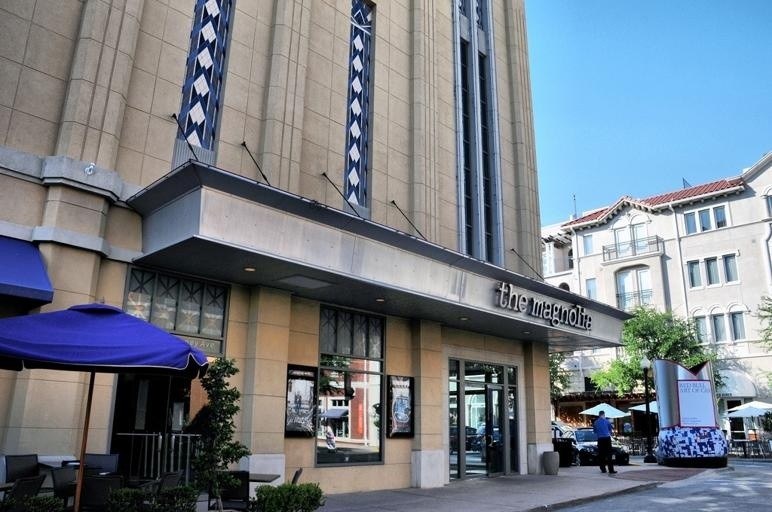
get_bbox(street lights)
[641,355,656,462]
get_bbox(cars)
[552,426,629,465]
[449,415,573,462]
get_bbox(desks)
[1,476,54,490]
[116,477,155,491]
[249,474,281,483]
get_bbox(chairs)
[52,466,77,508]
[5,454,39,482]
[4,474,48,501]
[291,468,303,485]
[139,473,177,492]
[85,454,119,476]
[86,476,122,504]
[208,470,256,511]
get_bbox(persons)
[593,411,617,474]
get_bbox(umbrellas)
[578,404,631,419]
[0,303,209,512]
[725,399,772,457]
